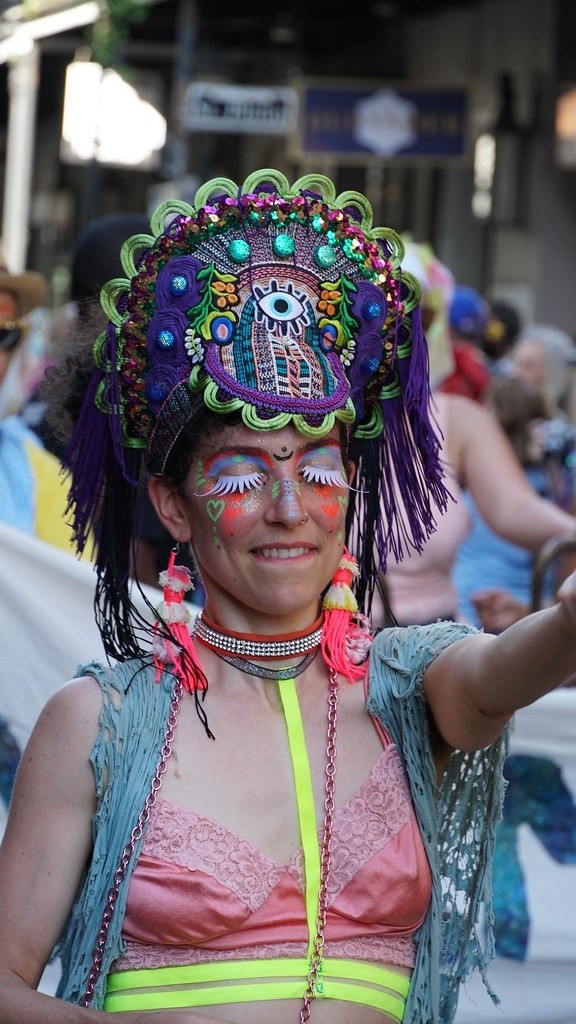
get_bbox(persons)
[1,170,576,1024]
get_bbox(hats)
[440,286,491,337]
[67,213,156,321]
[60,168,455,738]
[472,302,521,363]
[0,248,47,320]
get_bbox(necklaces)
[191,610,325,660]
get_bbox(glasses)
[0,325,25,351]
